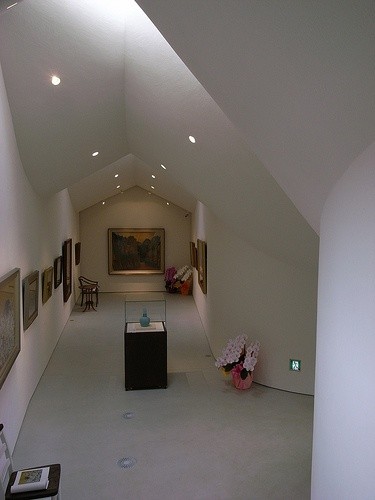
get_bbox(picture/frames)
[63,238,73,301]
[197,239,207,294]
[190,242,197,268]
[43,267,53,303]
[75,242,81,265]
[55,256,63,287]
[108,228,165,274]
[0,268,21,390]
[23,271,38,329]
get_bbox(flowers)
[173,265,192,287]
[164,267,176,289]
[215,335,260,380]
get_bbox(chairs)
[79,276,99,307]
[0,424,62,500]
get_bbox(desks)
[79,284,97,312]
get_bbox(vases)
[232,371,253,389]
[169,286,176,293]
[140,306,150,327]
[179,284,190,294]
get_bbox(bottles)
[140,313,150,327]
